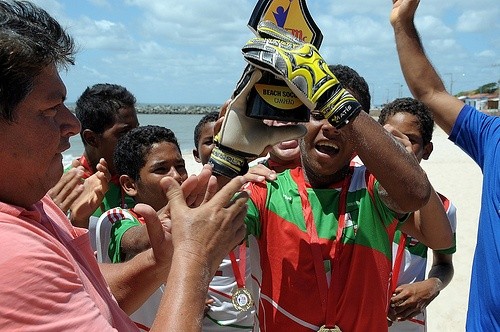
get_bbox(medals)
[318,325,342,332]
[232,289,254,312]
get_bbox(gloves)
[241,21,362,129]
[207,63,307,179]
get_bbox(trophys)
[246,0,323,122]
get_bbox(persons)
[0,0,248,332]
[46,83,301,332]
[390,0,500,332]
[373,98,457,332]
[208,20,431,332]
